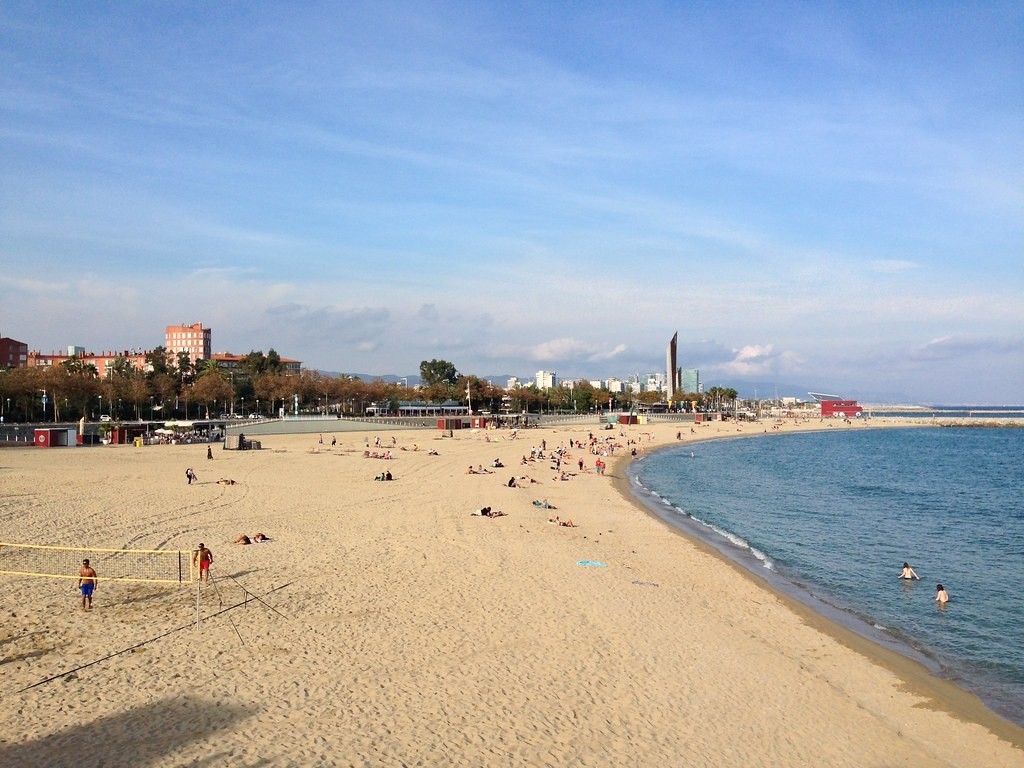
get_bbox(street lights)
[241,397,244,415]
[150,396,153,421]
[65,398,67,410]
[43,395,48,425]
[98,396,102,414]
[7,398,11,413]
[256,400,259,412]
[318,397,322,412]
[282,397,284,408]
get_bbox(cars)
[100,415,112,422]
[249,413,262,420]
[220,413,244,420]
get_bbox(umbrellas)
[155,428,174,434]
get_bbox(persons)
[898,562,920,581]
[732,416,871,432]
[936,583,949,602]
[193,543,213,582]
[219,478,234,485]
[451,427,695,527]
[186,467,194,484]
[319,433,438,482]
[208,445,212,459]
[237,533,266,544]
[78,559,97,610]
